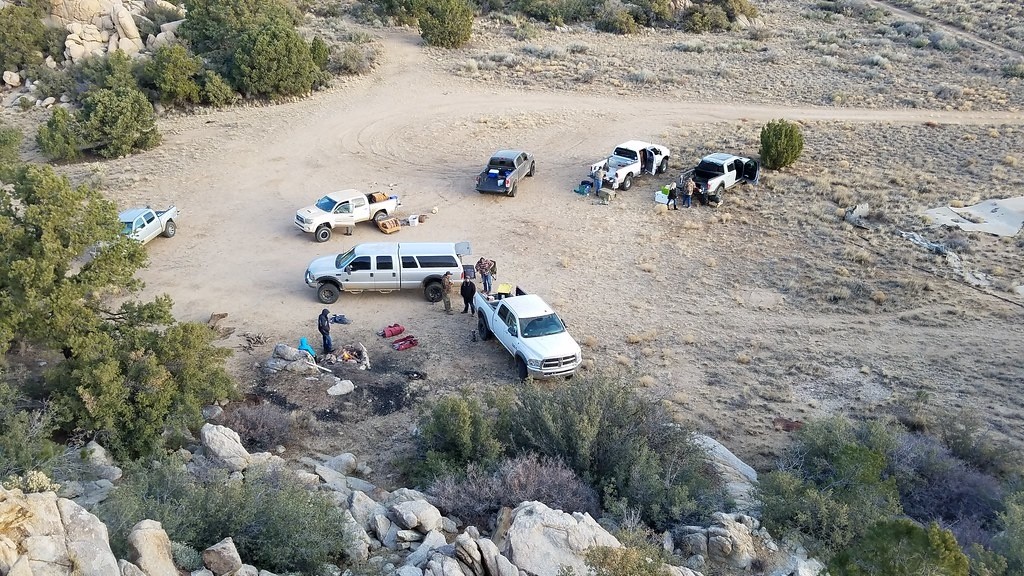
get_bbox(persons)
[318,309,333,352]
[346,226,353,235]
[594,166,605,196]
[461,276,476,317]
[441,271,454,315]
[611,173,619,197]
[666,182,678,210]
[475,257,494,294]
[682,177,696,208]
[298,337,318,365]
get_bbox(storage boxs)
[488,169,499,177]
[655,186,675,205]
[408,214,420,226]
[497,283,512,300]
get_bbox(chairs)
[490,260,497,281]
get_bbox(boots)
[668,205,670,210]
[674,205,678,210]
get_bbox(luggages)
[694,188,707,205]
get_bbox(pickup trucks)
[91,205,179,261]
[474,150,536,198]
[475,285,582,380]
[588,139,671,192]
[294,188,398,243]
[303,242,477,304]
[679,153,760,200]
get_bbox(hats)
[446,271,452,275]
[466,276,470,278]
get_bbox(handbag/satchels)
[675,198,678,205]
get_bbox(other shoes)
[472,313,474,317]
[683,204,686,206]
[481,290,487,293]
[688,206,691,209]
[461,310,467,314]
[486,291,491,294]
